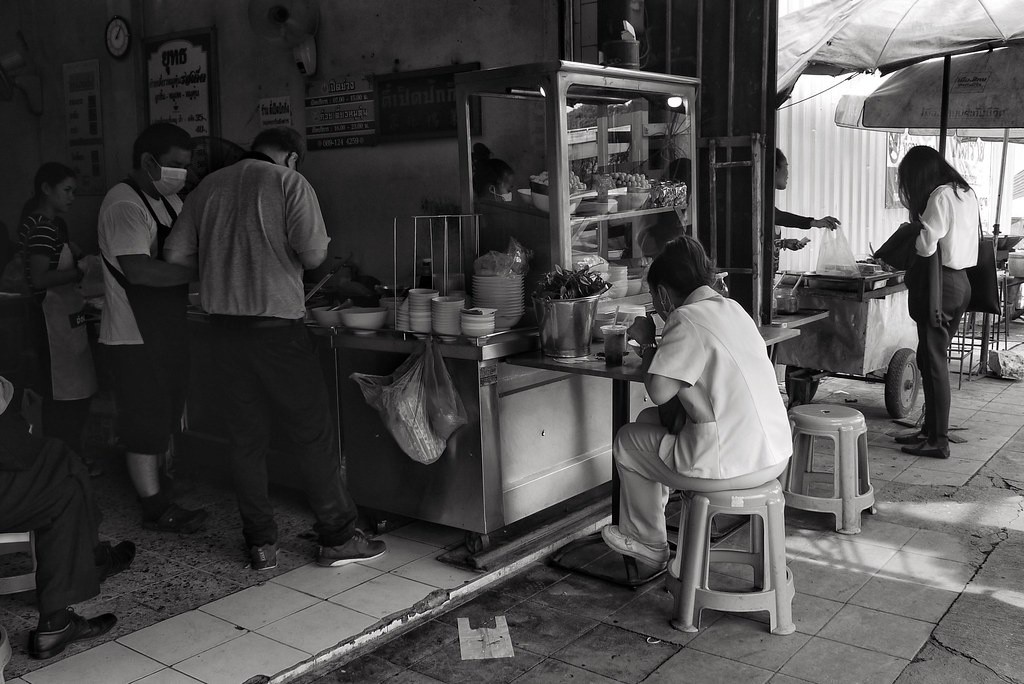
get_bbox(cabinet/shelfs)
[455,60,698,304]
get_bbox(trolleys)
[776,269,922,421]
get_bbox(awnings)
[776,0,866,111]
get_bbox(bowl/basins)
[395,180,651,344]
[342,308,388,334]
[312,306,361,327]
[628,337,663,358]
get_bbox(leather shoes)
[95,540,136,583]
[28,613,117,660]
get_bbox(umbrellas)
[807,0,1024,373]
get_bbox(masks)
[489,185,512,202]
[658,284,675,317]
[146,155,187,195]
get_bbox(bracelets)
[641,343,656,355]
[783,239,786,251]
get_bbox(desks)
[504,324,801,591]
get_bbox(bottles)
[772,287,797,318]
[602,40,640,70]
[711,271,729,297]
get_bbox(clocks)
[104,16,131,61]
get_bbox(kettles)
[600,325,626,367]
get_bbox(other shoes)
[901,440,950,458]
[895,430,928,445]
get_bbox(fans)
[0,49,41,100]
[248,0,319,75]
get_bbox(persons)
[16,160,100,473]
[773,148,841,278]
[547,154,586,182]
[602,235,793,570]
[98,121,209,533]
[471,158,515,202]
[0,377,137,660]
[895,145,979,458]
[163,128,387,571]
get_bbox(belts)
[210,319,304,329]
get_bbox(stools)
[667,480,796,636]
[779,403,878,534]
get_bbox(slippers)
[141,504,208,534]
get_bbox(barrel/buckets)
[532,295,600,358]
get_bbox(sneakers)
[601,524,670,570]
[316,528,387,567]
[249,540,281,571]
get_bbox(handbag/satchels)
[349,342,469,465]
[816,224,861,277]
[965,186,1002,316]
